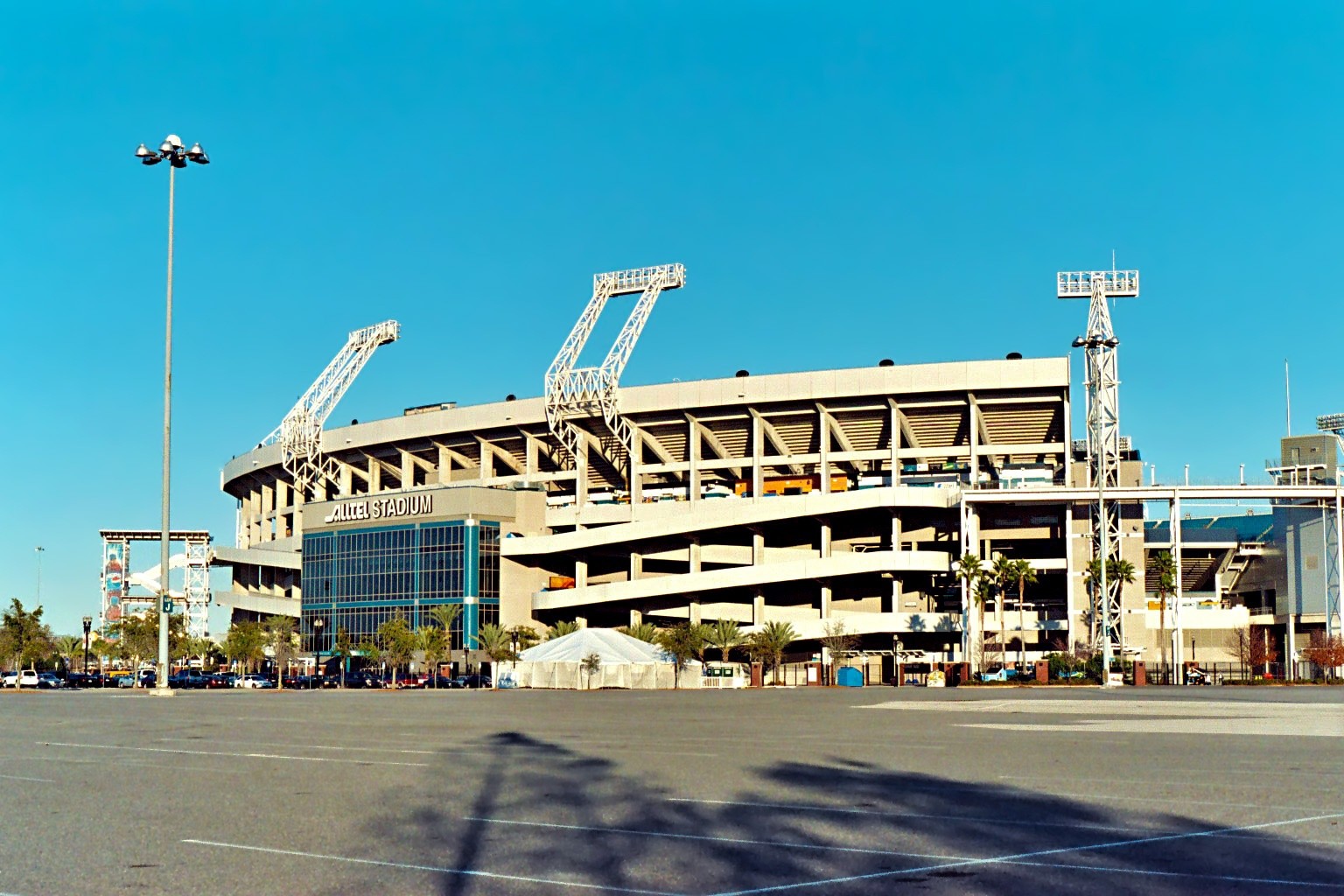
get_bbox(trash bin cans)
[702,663,742,688]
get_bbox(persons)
[1218,673,1223,683]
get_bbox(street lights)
[82,617,92,675]
[133,131,210,694]
[892,635,899,686]
[34,546,45,607]
[1072,334,1120,684]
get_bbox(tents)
[492,628,702,690]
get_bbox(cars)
[0,668,493,690]
